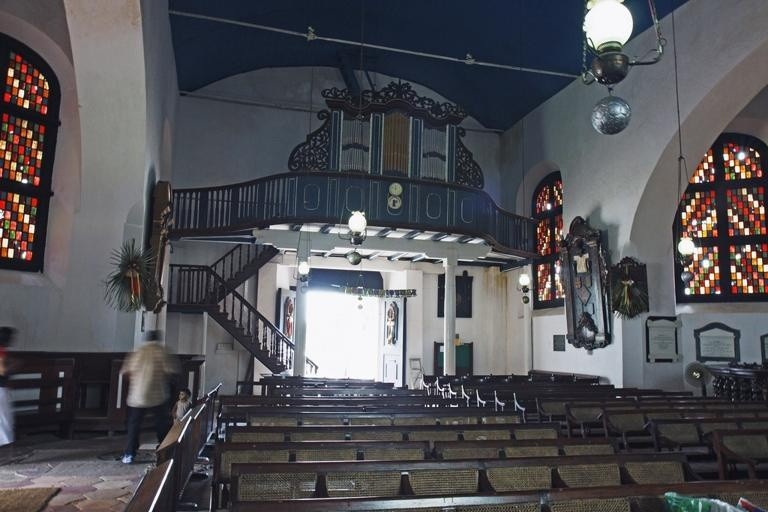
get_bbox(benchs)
[213,370,768,511]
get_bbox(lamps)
[577,0,667,137]
[337,206,371,267]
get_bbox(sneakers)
[122,454,133,464]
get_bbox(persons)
[0,326,21,446]
[120,329,182,463]
[171,388,192,425]
